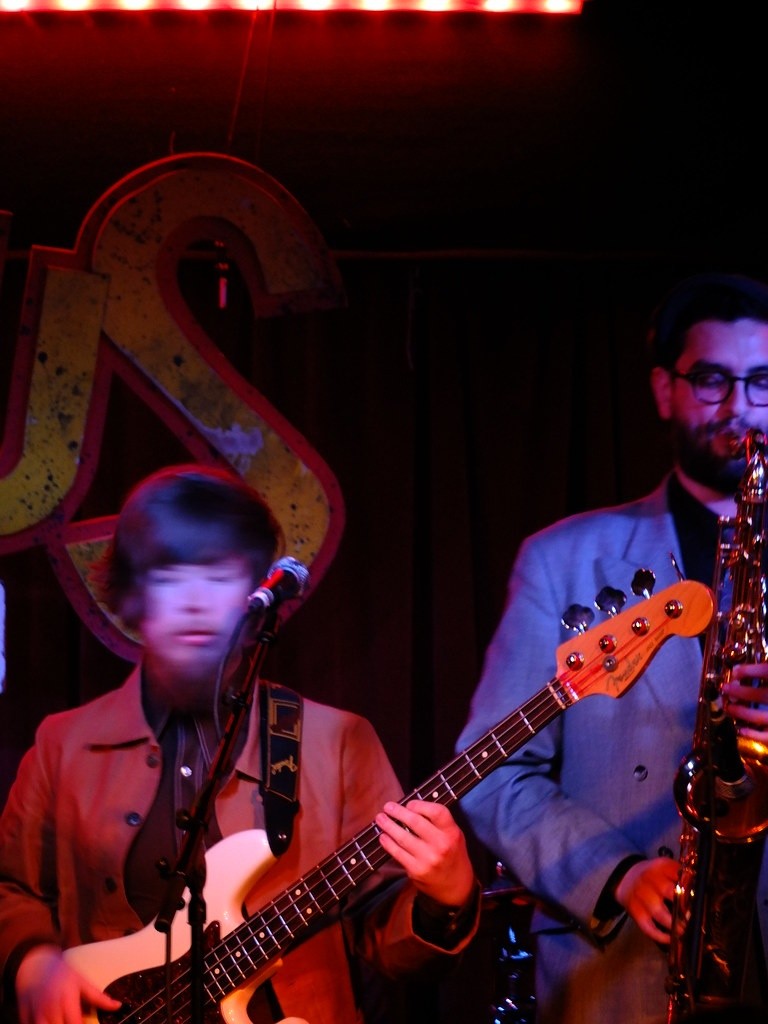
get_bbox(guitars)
[63,553,717,1024]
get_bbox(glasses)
[666,369,768,407]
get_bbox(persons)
[0,460,479,1023]
[453,290,768,1023]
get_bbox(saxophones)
[659,427,766,1023]
